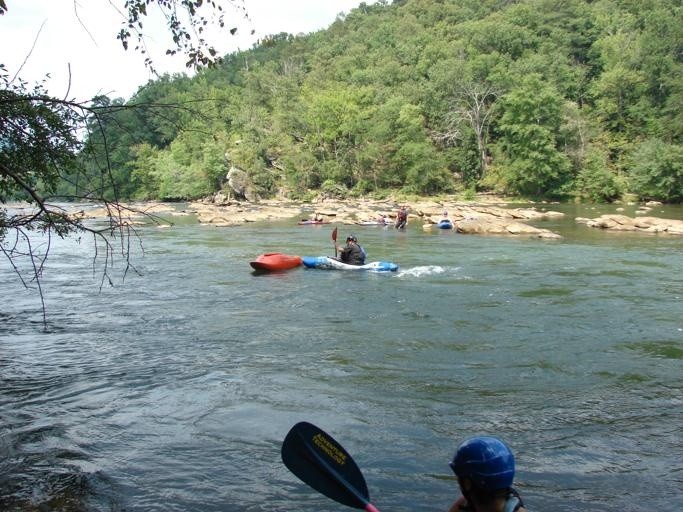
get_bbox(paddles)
[332,226,337,257]
[281,421,379,512]
[302,219,309,221]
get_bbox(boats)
[251,253,304,272]
[358,217,394,226]
[298,220,332,225]
[304,255,398,273]
[438,223,453,230]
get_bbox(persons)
[394,206,408,229]
[446,436,528,512]
[376,214,388,224]
[440,211,454,228]
[315,213,322,221]
[336,235,366,266]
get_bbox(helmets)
[347,235,357,242]
[449,436,515,490]
